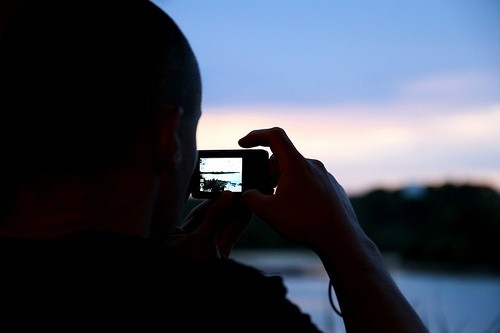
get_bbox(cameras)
[192,149,273,199]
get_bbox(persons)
[0,0,430,333]
